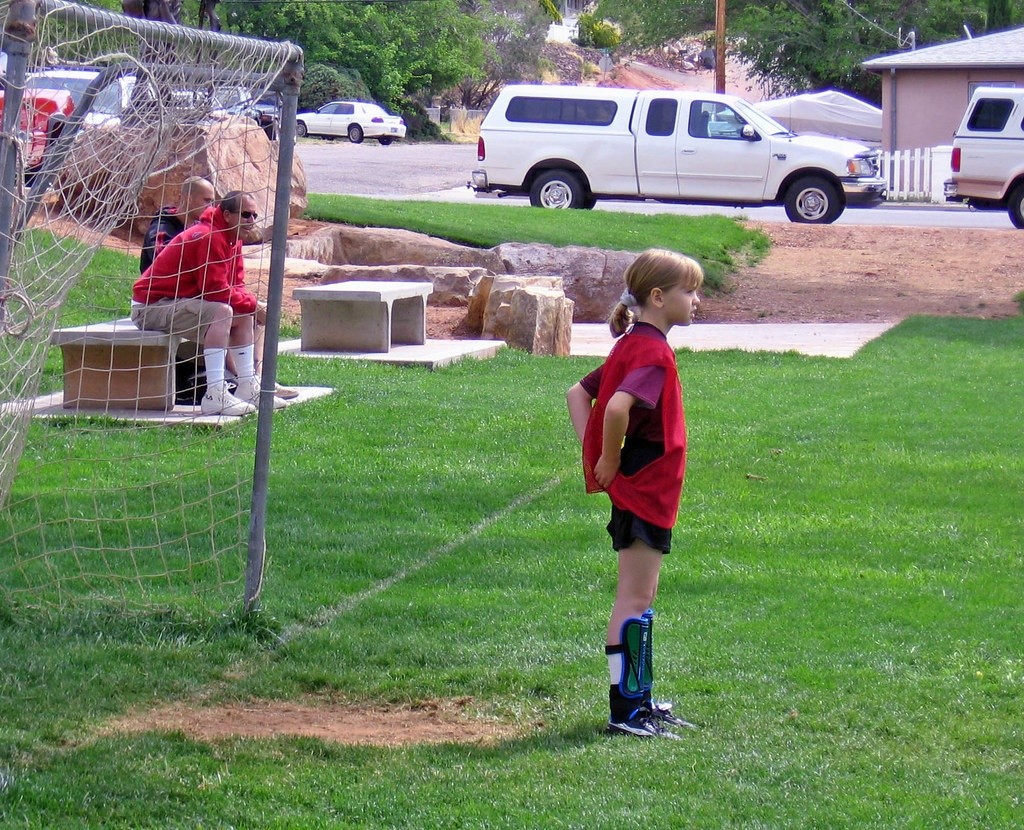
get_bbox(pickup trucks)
[943,86,1024,229]
[466,85,888,225]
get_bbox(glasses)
[229,210,258,220]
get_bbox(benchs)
[50,314,205,412]
[291,280,433,353]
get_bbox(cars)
[296,101,406,145]
[1,71,283,171]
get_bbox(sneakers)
[605,703,693,741]
[200,388,256,417]
[234,388,288,409]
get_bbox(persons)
[565,249,705,745]
[130,190,287,415]
[139,175,299,399]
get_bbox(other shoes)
[276,383,300,399]
